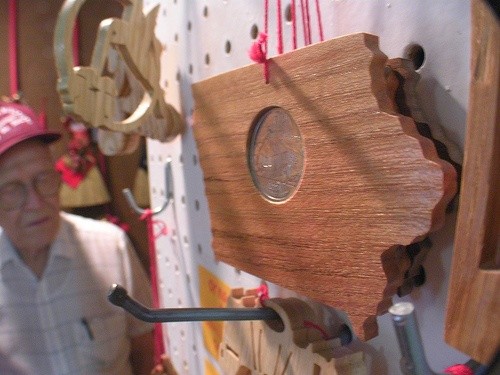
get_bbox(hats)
[0,100,64,156]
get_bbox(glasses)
[1,171,64,210]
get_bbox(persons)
[0,100,171,375]
